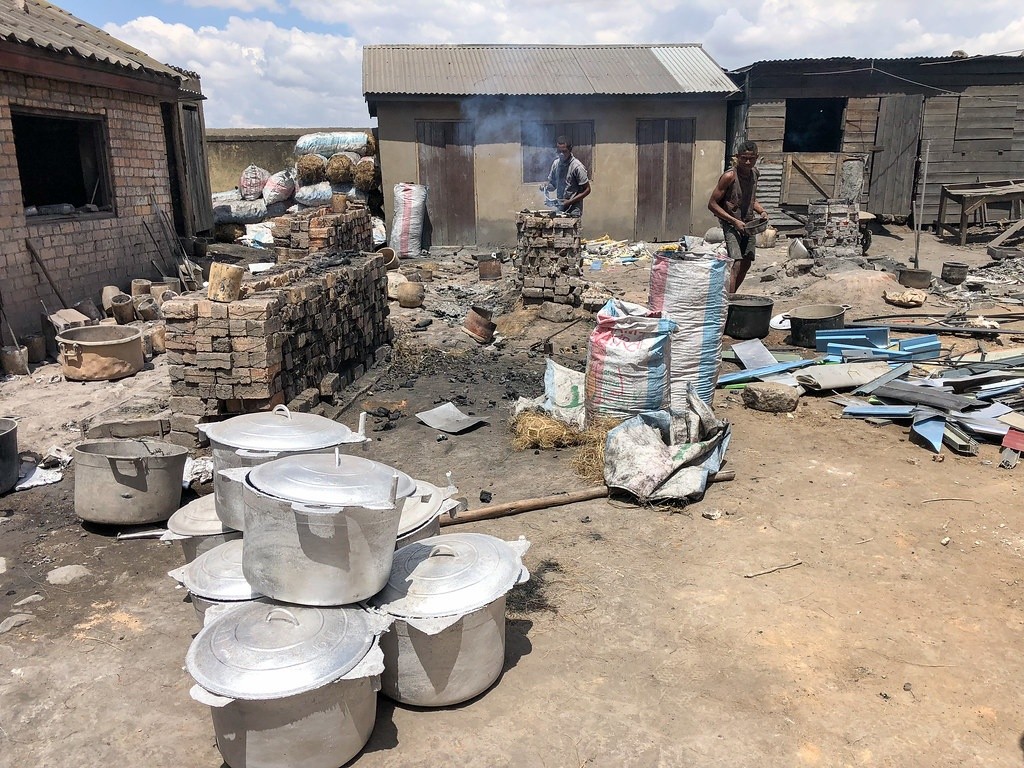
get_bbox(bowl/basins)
[743,218,768,234]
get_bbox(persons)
[538,134,591,218]
[707,141,769,294]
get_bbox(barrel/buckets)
[941,262,969,285]
[195,403,373,532]
[159,492,243,564]
[728,293,775,338]
[54,325,144,380]
[73,437,188,525]
[782,304,852,347]
[0,415,20,494]
[899,269,931,288]
[185,596,382,768]
[217,448,416,606]
[395,479,461,552]
[367,533,532,706]
